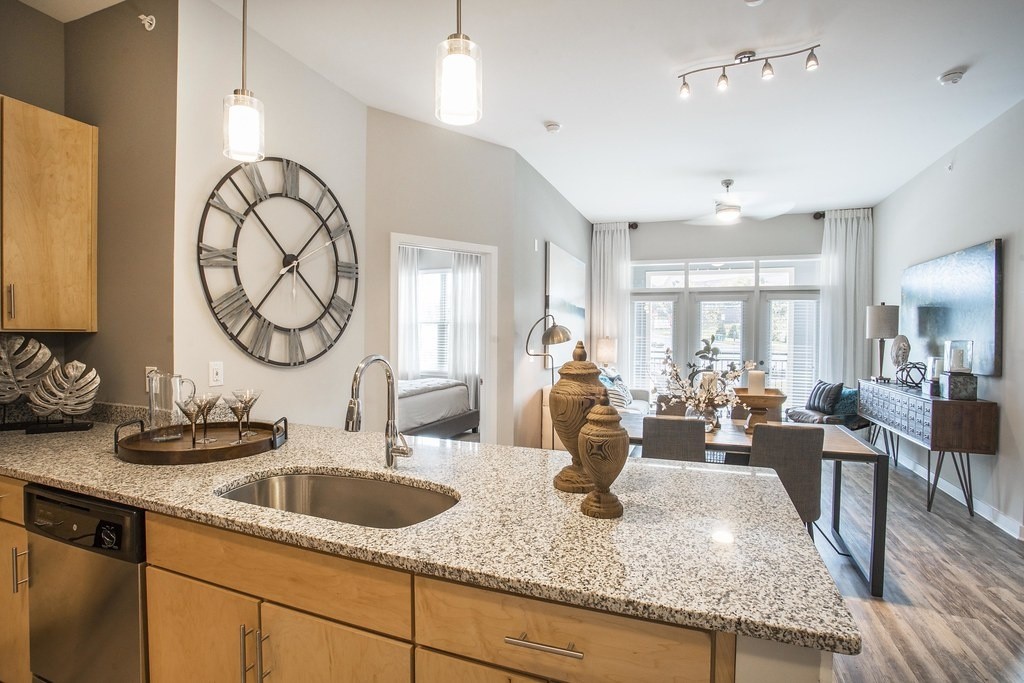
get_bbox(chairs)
[642,416,706,462]
[748,423,825,542]
[656,395,687,416]
[785,386,876,431]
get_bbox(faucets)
[344,353,413,469]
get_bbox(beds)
[398,378,479,438]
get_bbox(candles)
[747,370,765,394]
[934,360,943,377]
[952,349,964,368]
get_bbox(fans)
[682,177,796,226]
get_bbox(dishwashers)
[11,483,148,683]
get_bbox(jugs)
[146,370,196,442]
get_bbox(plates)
[891,335,910,367]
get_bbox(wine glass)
[187,393,223,444]
[174,398,211,448]
[232,390,263,437]
[223,396,255,446]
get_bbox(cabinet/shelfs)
[0,94,98,332]
[0,478,736,683]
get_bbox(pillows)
[606,379,633,409]
[804,379,844,416]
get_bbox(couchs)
[542,383,650,452]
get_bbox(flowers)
[645,333,757,411]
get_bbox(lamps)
[222,0,265,162]
[677,44,821,97]
[434,0,482,126]
[596,336,618,367]
[866,302,899,383]
[716,205,740,221]
[526,314,572,450]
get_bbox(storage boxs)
[921,381,939,396]
[939,374,977,400]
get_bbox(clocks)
[197,156,359,365]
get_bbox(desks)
[856,379,998,517]
[617,413,889,598]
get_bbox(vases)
[685,405,717,432]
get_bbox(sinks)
[210,462,462,530]
